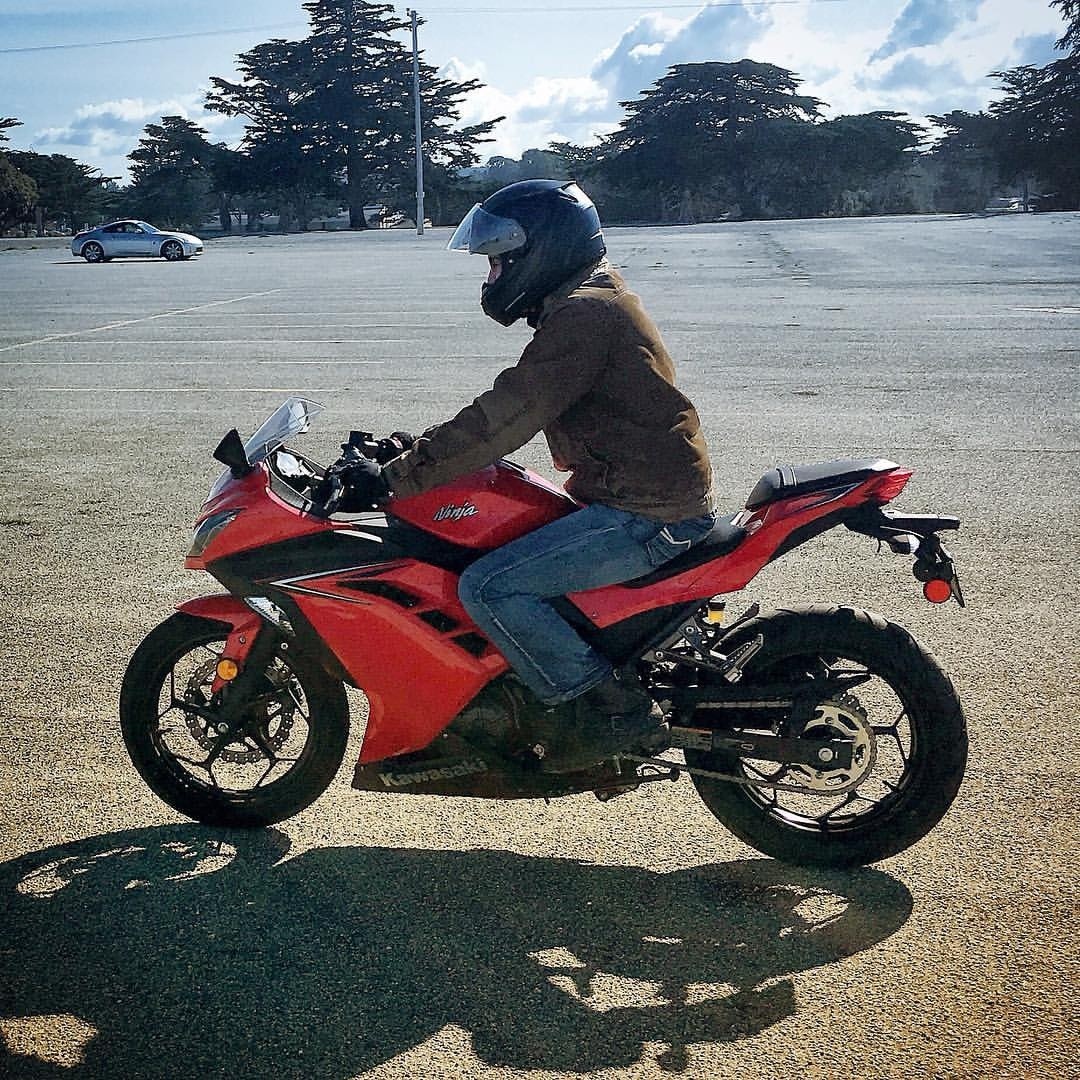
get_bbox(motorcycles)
[116,394,970,873]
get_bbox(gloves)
[343,459,388,500]
[379,431,417,463]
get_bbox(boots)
[531,669,672,774]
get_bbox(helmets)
[472,183,607,326]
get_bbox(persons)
[323,178,717,774]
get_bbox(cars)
[983,197,1025,214]
[47,230,66,237]
[72,220,204,264]
